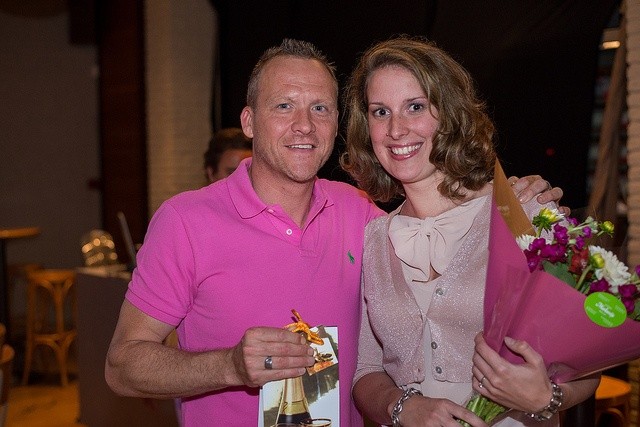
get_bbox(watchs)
[525,382,564,422]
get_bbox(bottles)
[275,376,313,427]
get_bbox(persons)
[204,128,253,184]
[340,40,602,427]
[105,42,571,427]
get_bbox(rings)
[265,356,272,369]
[479,376,486,387]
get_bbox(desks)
[0,226,42,386]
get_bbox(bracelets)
[391,387,423,427]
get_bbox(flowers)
[458,207,640,426]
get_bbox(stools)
[20,264,80,386]
